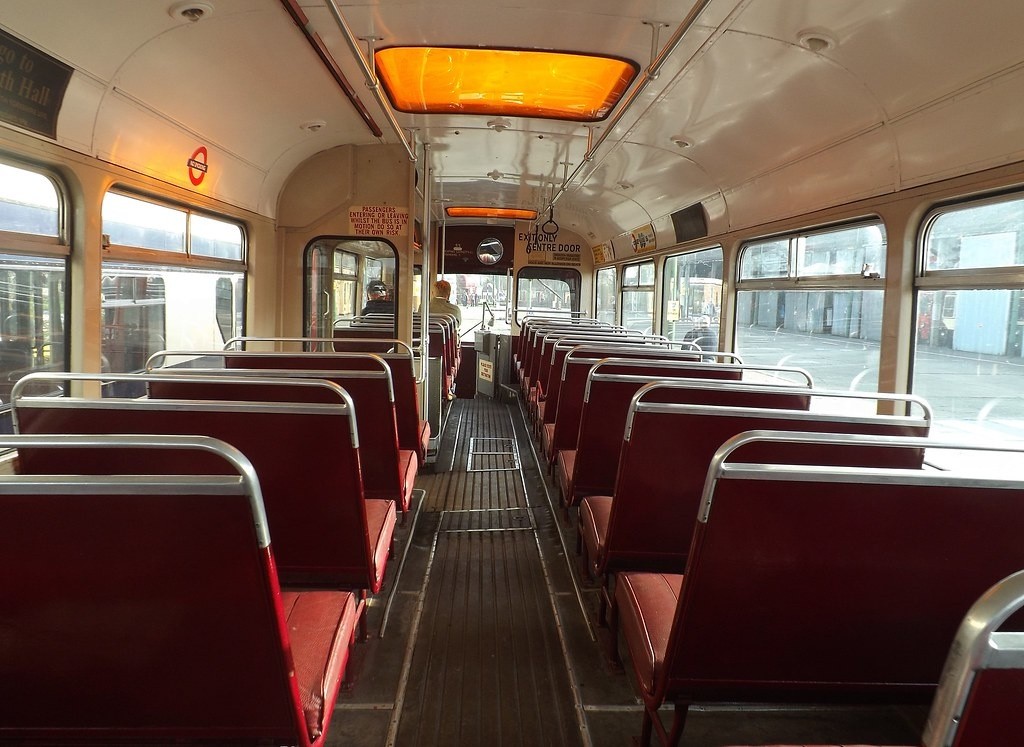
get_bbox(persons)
[361,280,395,323]
[418,279,461,328]
[681,314,719,360]
[704,300,715,323]
[462,290,477,307]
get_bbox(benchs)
[0,313,460,747]
[515,315,1024,747]
